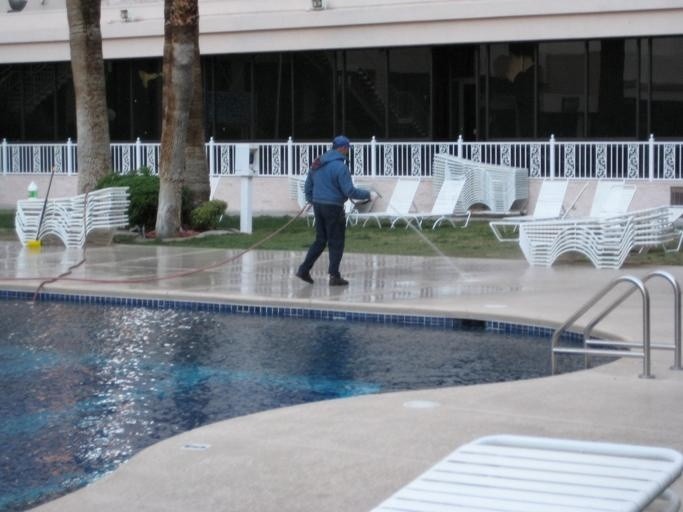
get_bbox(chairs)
[370,434,683,512]
[289,152,529,231]
[489,176,683,269]
[14,187,131,248]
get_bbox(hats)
[333,136,352,147]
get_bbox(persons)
[297,135,380,285]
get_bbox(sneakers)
[329,277,348,286]
[296,271,313,284]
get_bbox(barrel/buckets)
[27,180,38,198]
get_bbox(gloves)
[369,191,379,201]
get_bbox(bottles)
[27,182,38,201]
